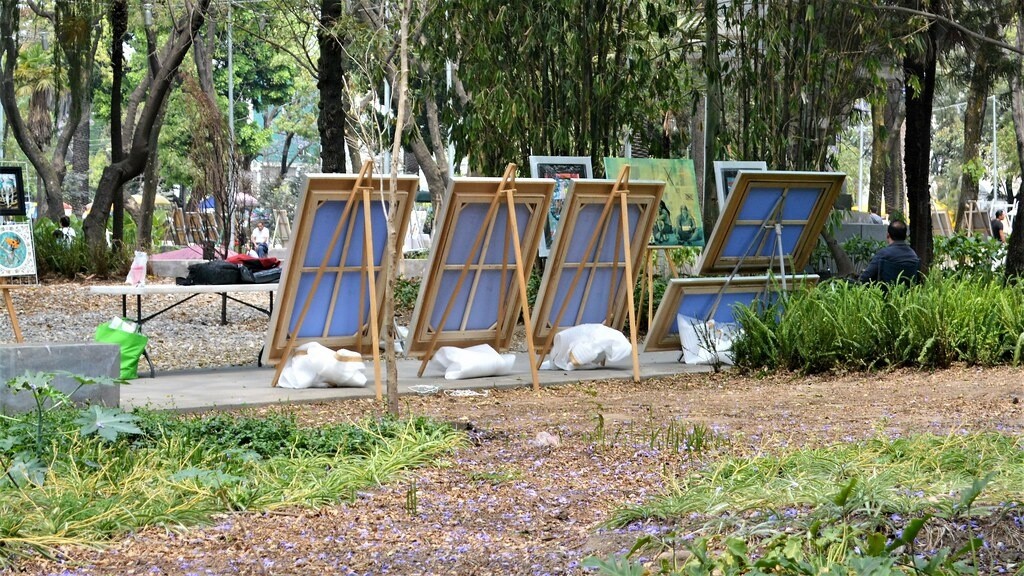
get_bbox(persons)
[250,222,269,258]
[862,219,920,286]
[59,216,77,244]
[991,209,1006,243]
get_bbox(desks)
[90,284,280,379]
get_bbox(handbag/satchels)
[95,317,148,380]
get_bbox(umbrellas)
[196,191,260,208]
